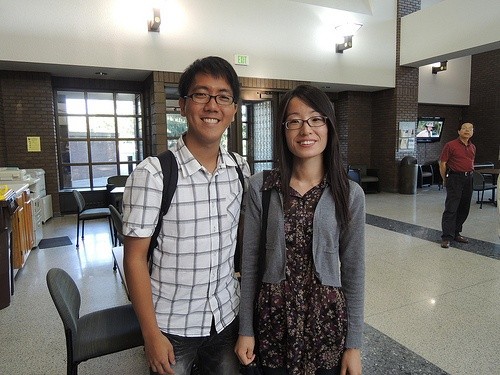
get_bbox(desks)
[475,169,499,208]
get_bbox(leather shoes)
[455,236,468,243]
[441,240,450,248]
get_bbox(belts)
[449,171,474,176]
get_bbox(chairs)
[73,177,125,270]
[472,170,497,209]
[419,164,434,188]
[45,268,145,375]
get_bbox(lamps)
[334,22,363,54]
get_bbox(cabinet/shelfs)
[0,185,35,309]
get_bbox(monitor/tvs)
[416,117,445,142]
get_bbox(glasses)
[283,115,329,130]
[185,93,237,106]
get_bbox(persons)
[234,85,366,375]
[417,122,435,137]
[439,121,476,248]
[122,57,251,375]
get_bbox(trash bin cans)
[399,155,418,194]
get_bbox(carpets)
[38,236,73,248]
